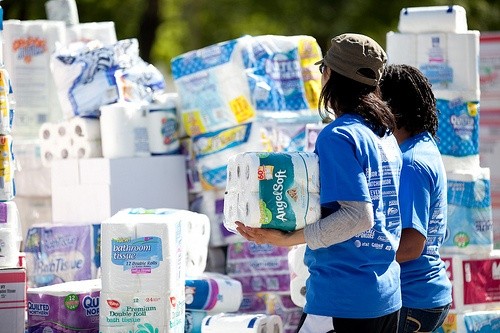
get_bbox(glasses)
[319,65,329,77]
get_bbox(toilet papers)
[0,0,499,333]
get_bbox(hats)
[314,33,388,86]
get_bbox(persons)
[235,33,404,333]
[381,64,453,333]
